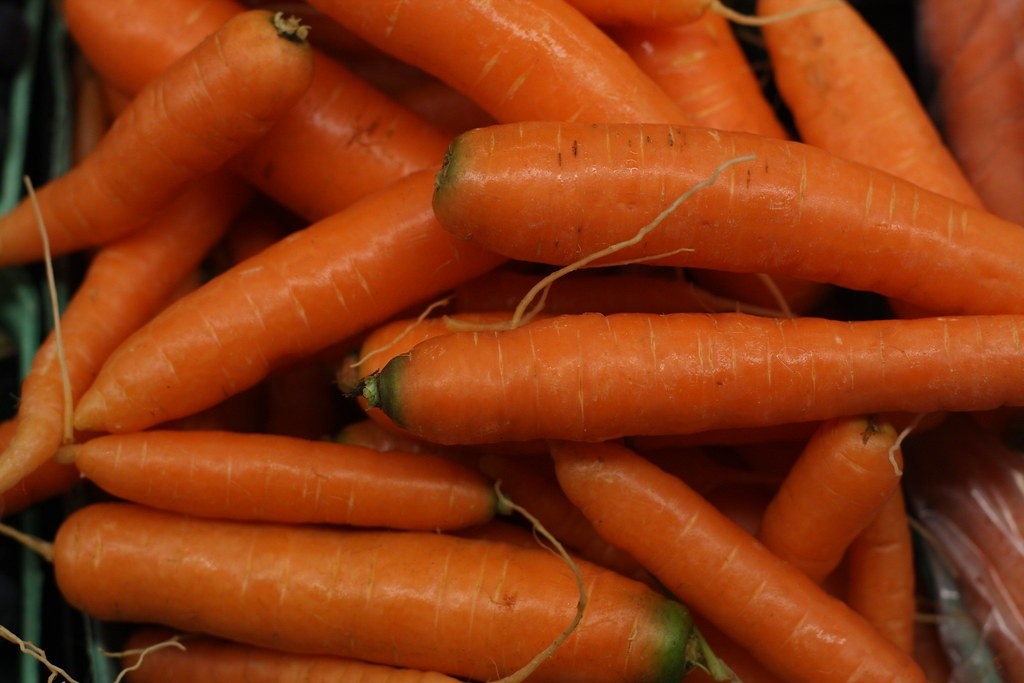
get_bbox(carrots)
[2,0,1024,681]
